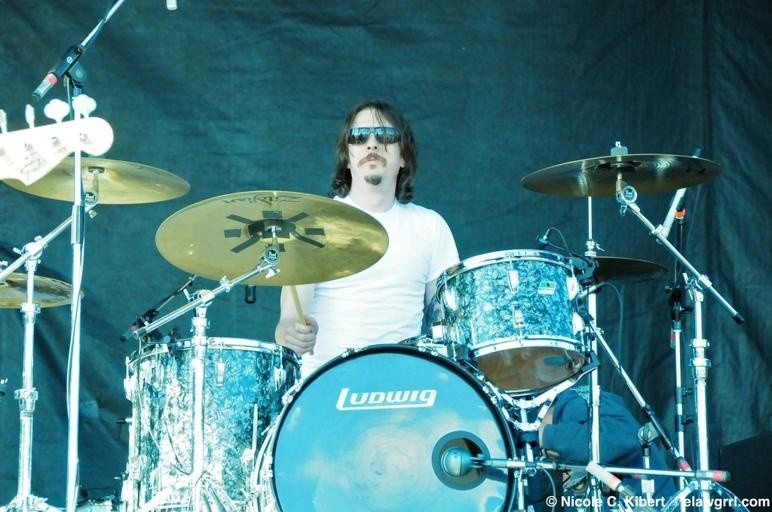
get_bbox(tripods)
[135,340,242,512]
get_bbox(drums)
[251,345,522,511]
[434,249,591,397]
[122,337,301,510]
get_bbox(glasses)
[346,126,397,144]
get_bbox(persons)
[273,99,462,380]
[537,386,677,511]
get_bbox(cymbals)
[0,156,191,205]
[522,153,722,196]
[583,256,667,283]
[155,190,389,287]
[0,270,84,308]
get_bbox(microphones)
[583,457,662,512]
[656,188,688,247]
